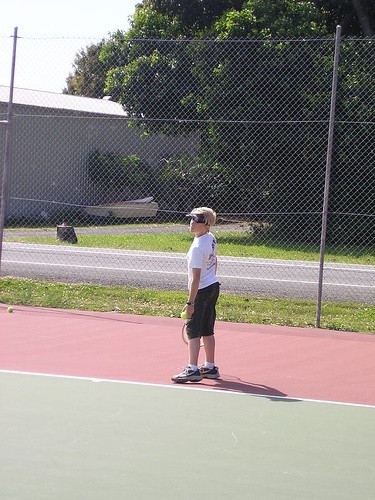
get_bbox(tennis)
[182,312,187,319]
[7,307,13,313]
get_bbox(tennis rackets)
[182,319,204,348]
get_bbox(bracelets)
[186,302,195,306]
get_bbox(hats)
[186,207,216,226]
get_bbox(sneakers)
[199,366,220,378]
[171,366,203,383]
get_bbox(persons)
[171,207,222,383]
